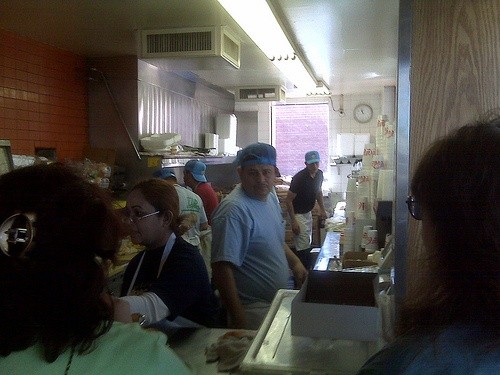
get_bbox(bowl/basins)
[140,132,181,152]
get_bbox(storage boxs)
[290,270,382,343]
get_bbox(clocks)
[353,103,373,124]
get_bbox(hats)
[305,151,320,164]
[184,160,207,182]
[151,168,177,179]
[237,143,281,177]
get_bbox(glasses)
[406,195,439,220]
[122,210,160,224]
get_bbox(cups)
[343,114,393,253]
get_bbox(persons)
[183,160,219,221]
[0,155,194,375]
[286,150,327,271]
[210,142,307,330]
[273,165,283,185]
[105,177,223,328]
[150,169,207,256]
[359,108,500,375]
